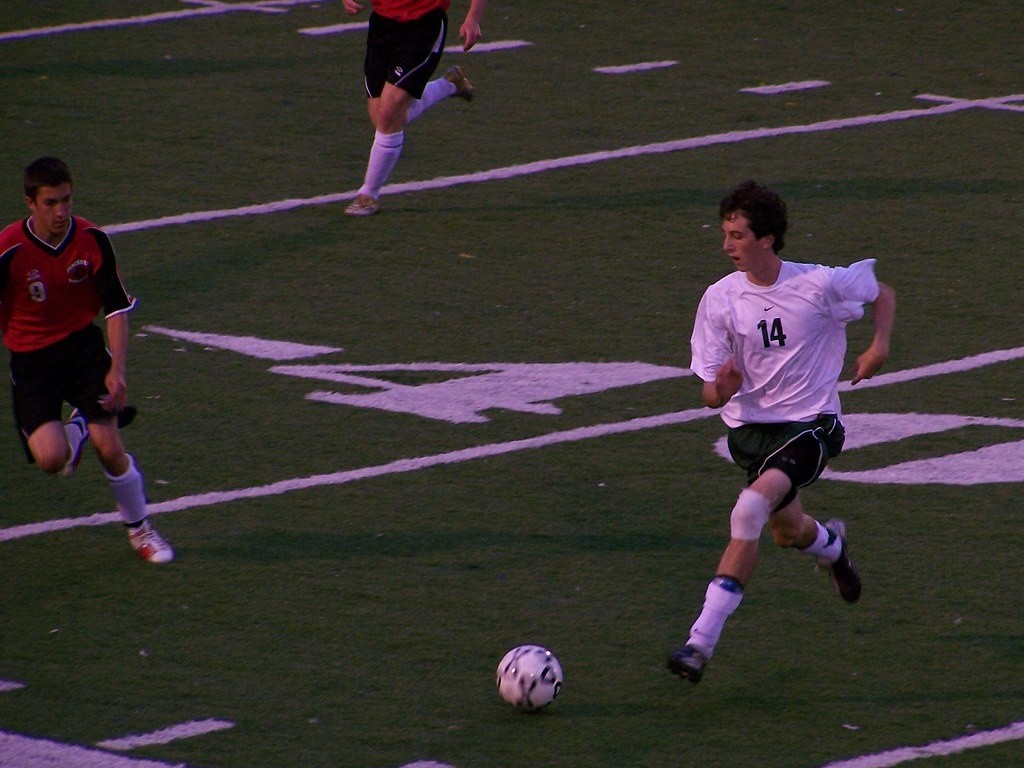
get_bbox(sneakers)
[342,193,381,216]
[127,518,173,564]
[664,645,709,687]
[62,407,90,478]
[814,517,861,604]
[443,63,474,104]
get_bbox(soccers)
[497,644,564,711]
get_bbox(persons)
[345,1,488,216]
[663,180,896,684]
[0,158,176,562]
